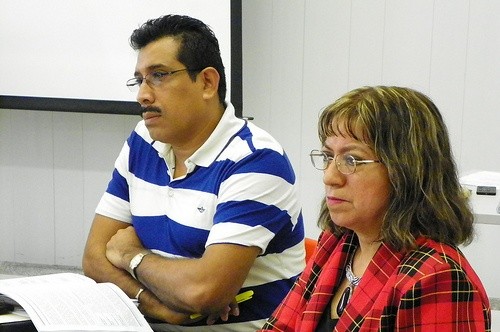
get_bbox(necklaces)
[335,241,363,316]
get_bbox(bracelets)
[132,285,147,302]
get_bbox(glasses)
[126,66,203,90]
[309,147,392,175]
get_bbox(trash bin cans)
[450,170,500,312]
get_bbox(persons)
[257,85,492,331]
[82,14,306,332]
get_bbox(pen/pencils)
[188,289,255,321]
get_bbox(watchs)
[128,250,155,281]
